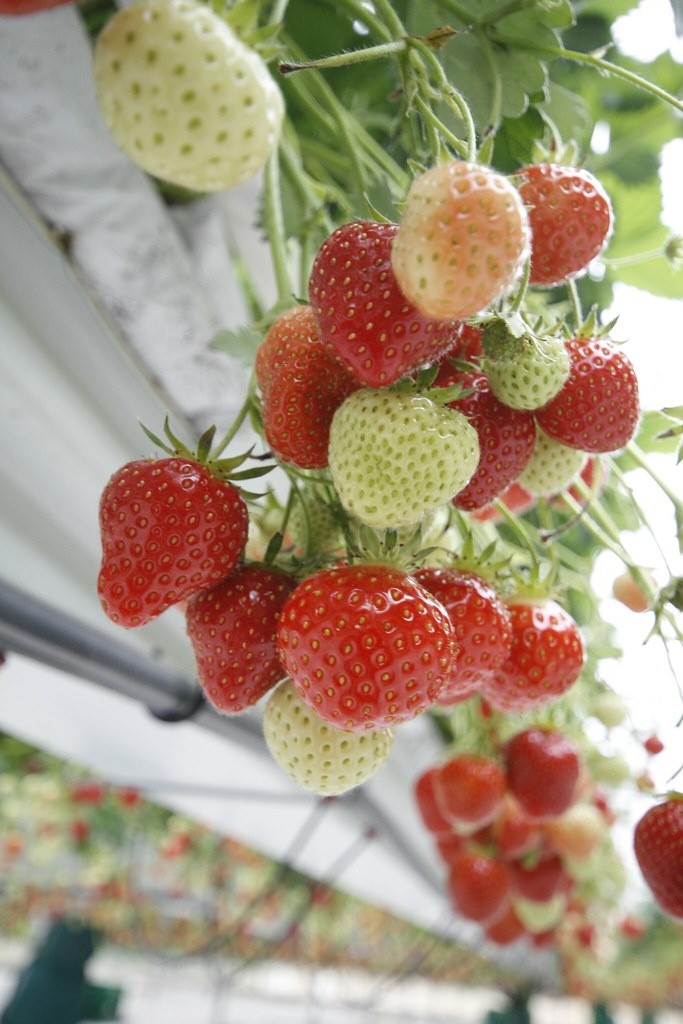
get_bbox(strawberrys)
[91,0,682,940]
